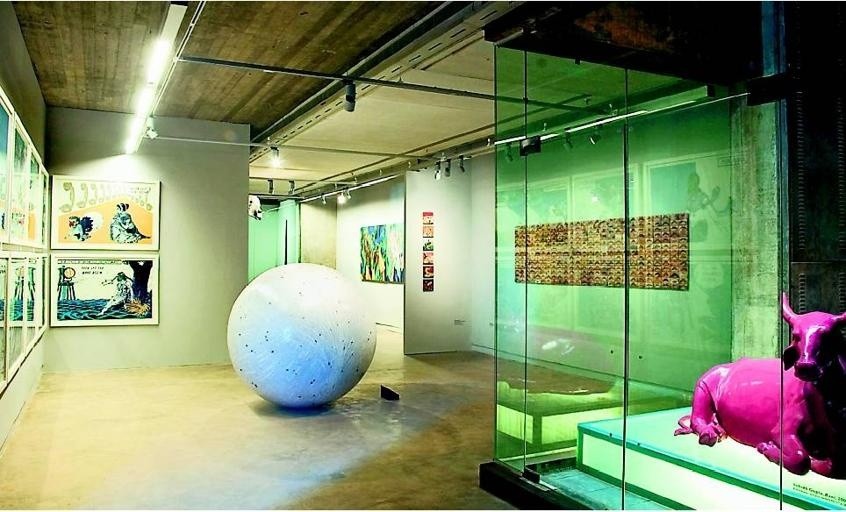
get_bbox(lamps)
[434,153,467,181]
[266,179,296,195]
[344,80,357,112]
[562,124,603,152]
[504,141,514,164]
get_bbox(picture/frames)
[1,251,50,394]
[50,175,161,252]
[0,86,51,251]
[50,250,160,328]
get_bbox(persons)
[109,201,144,246]
[95,271,135,317]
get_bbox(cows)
[674,291,846,479]
